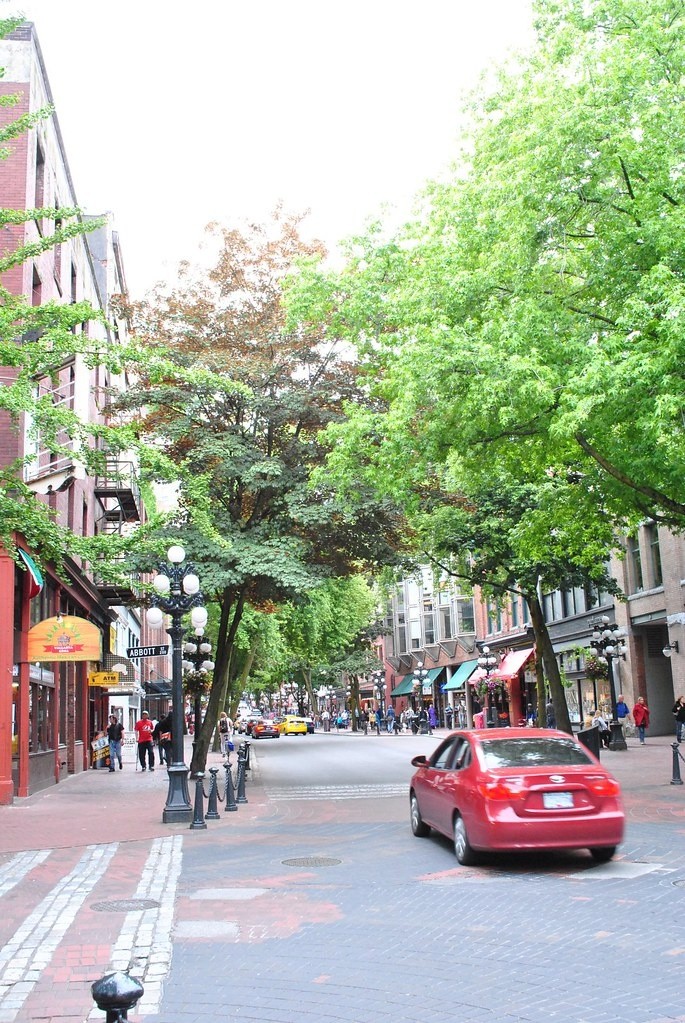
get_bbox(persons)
[159,711,173,766]
[672,696,685,743]
[585,710,612,748]
[303,699,465,734]
[218,712,233,757]
[546,699,555,728]
[134,711,154,772]
[616,695,631,739]
[152,717,159,727]
[154,714,167,766]
[107,714,124,772]
[633,697,650,744]
[525,703,537,724]
[473,698,482,713]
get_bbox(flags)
[18,548,42,599]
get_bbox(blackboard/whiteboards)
[121,731,138,764]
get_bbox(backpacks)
[219,719,228,733]
[594,718,603,732]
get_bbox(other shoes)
[142,767,146,772]
[640,741,646,745]
[120,763,123,770]
[678,740,681,743]
[604,742,609,748]
[223,754,225,757]
[109,767,116,772]
[149,766,154,771]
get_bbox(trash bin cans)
[577,725,600,760]
[411,716,418,732]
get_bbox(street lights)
[145,545,213,824]
[590,615,628,752]
[477,646,498,728]
[410,661,429,734]
[372,671,387,730]
[325,685,336,728]
[181,624,214,771]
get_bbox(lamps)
[662,641,679,657]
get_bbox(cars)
[232,700,285,736]
[303,717,315,734]
[251,719,280,739]
[277,715,308,736]
[409,727,626,867]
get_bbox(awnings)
[443,660,479,690]
[390,674,414,696]
[498,648,534,680]
[423,667,444,687]
[403,683,414,694]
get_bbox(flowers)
[412,683,422,697]
[584,657,608,679]
[473,675,502,697]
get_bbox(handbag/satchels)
[673,703,679,718]
[387,715,393,721]
